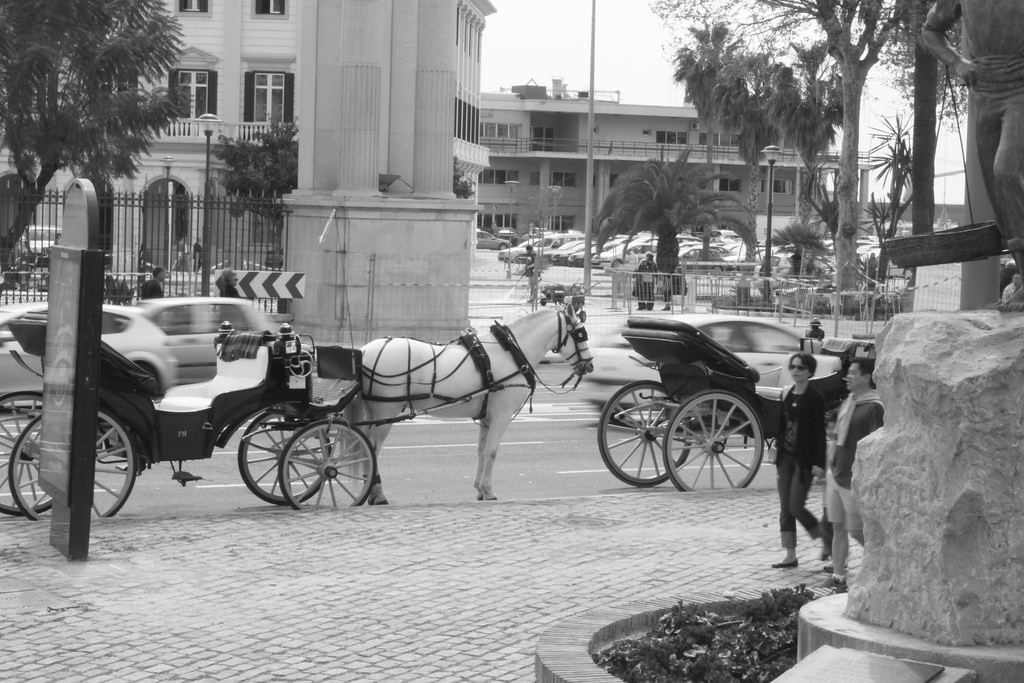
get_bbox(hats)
[809,318,822,326]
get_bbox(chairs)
[730,331,751,352]
[669,276,696,312]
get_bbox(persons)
[791,251,816,275]
[758,256,766,298]
[193,237,203,272]
[856,253,877,290]
[633,252,658,311]
[770,352,884,589]
[524,245,540,302]
[999,260,1021,301]
[219,270,240,298]
[141,267,166,300]
[661,273,672,311]
[921,0,1024,313]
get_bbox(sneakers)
[824,561,849,572]
[813,577,848,594]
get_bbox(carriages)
[0,291,596,525]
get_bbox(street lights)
[504,179,519,280]
[193,112,222,299]
[759,144,784,312]
[550,185,562,234]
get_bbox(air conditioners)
[690,121,700,129]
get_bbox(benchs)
[264,339,318,364]
[755,353,842,400]
[156,340,268,410]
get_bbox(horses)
[344,293,595,504]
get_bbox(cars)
[0,301,181,407]
[9,223,62,271]
[475,227,917,281]
[131,297,279,388]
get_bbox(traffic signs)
[212,268,305,299]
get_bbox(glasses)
[788,364,809,372]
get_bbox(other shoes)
[821,532,833,561]
[771,558,799,568]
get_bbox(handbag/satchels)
[769,446,778,464]
